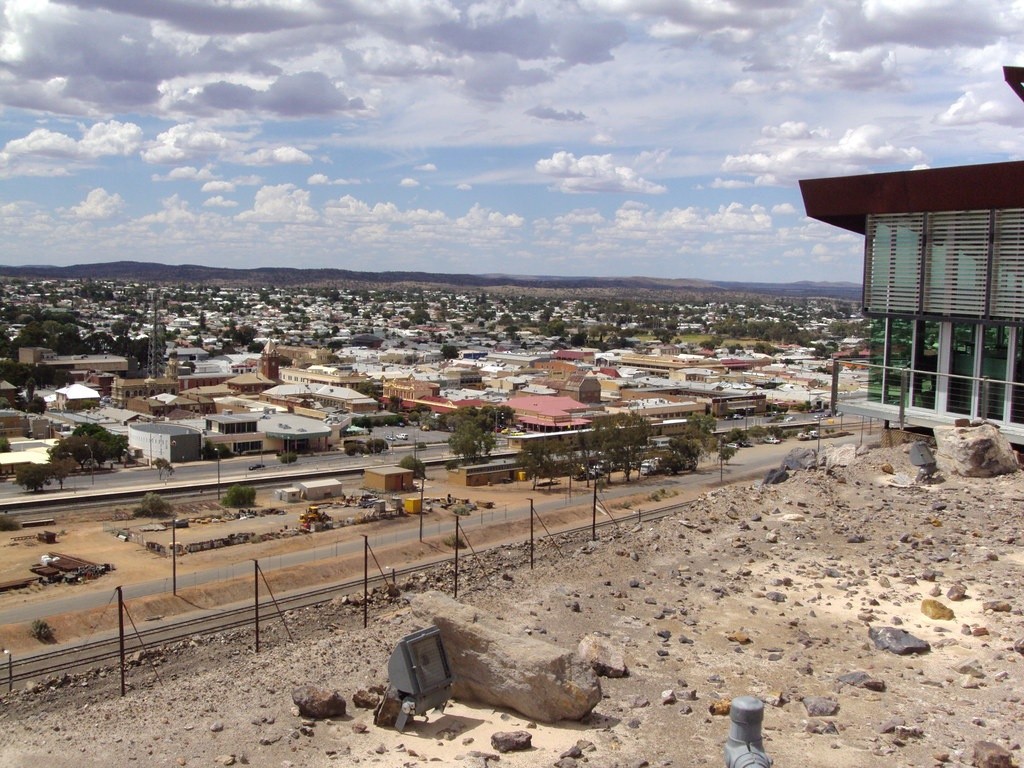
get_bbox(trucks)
[641,457,664,476]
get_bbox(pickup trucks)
[764,437,780,444]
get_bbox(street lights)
[85,444,94,485]
[214,447,221,501]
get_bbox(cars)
[249,464,265,470]
[795,431,819,440]
[396,433,408,440]
[501,428,511,434]
[785,417,796,422]
[385,435,396,441]
[727,443,740,448]
[396,422,405,427]
[812,415,820,421]
[516,425,526,432]
[724,416,732,420]
[825,413,831,417]
[733,414,743,419]
[575,459,641,481]
[740,441,753,447]
[836,411,843,417]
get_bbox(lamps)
[387,626,453,734]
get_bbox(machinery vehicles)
[300,506,332,525]
